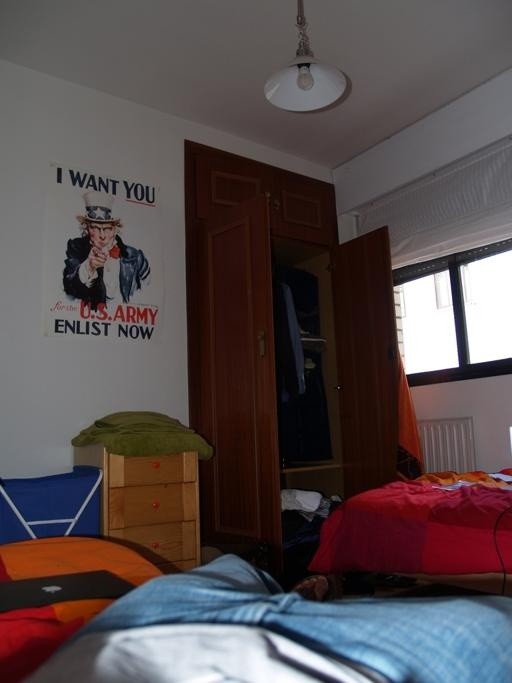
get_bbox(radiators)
[417,415,476,473]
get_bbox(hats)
[83,192,120,223]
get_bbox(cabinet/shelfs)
[186,138,400,574]
[74,444,202,574]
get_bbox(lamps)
[265,0,346,111]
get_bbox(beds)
[342,470,510,596]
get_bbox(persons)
[20,552,512,682]
[56,187,153,315]
